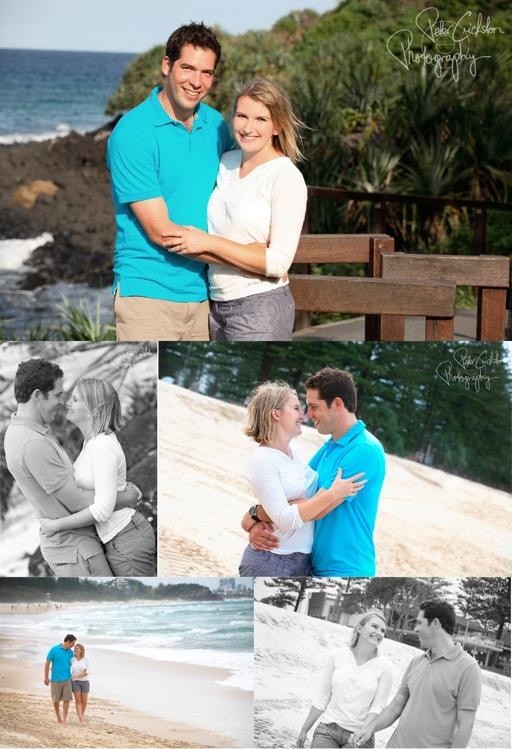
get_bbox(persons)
[242,366,387,576]
[4,359,155,577]
[295,598,481,748]
[106,20,308,341]
[44,633,90,724]
